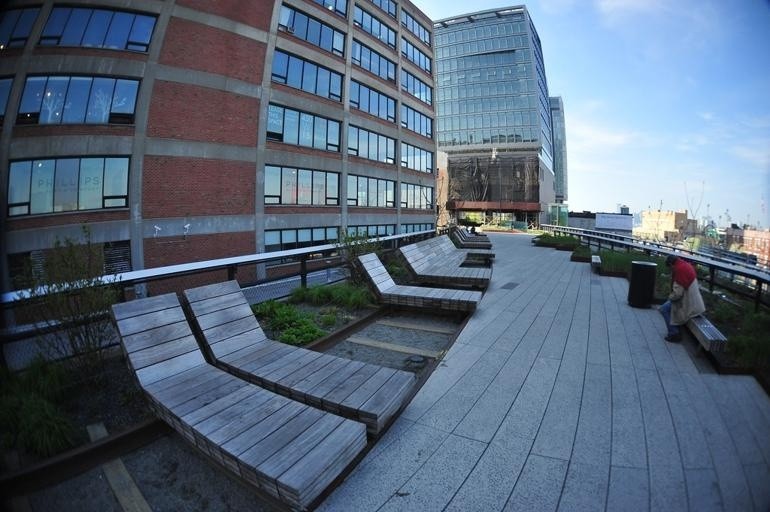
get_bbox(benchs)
[683,313,729,359]
[591,254,602,274]
[530,238,540,247]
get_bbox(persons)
[465,222,470,232]
[658,253,708,344]
[470,224,477,234]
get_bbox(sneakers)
[664,333,683,343]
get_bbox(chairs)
[355,253,482,315]
[110,293,369,511]
[399,234,496,286]
[451,228,492,249]
[183,281,415,441]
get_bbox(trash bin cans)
[628,260,658,309]
